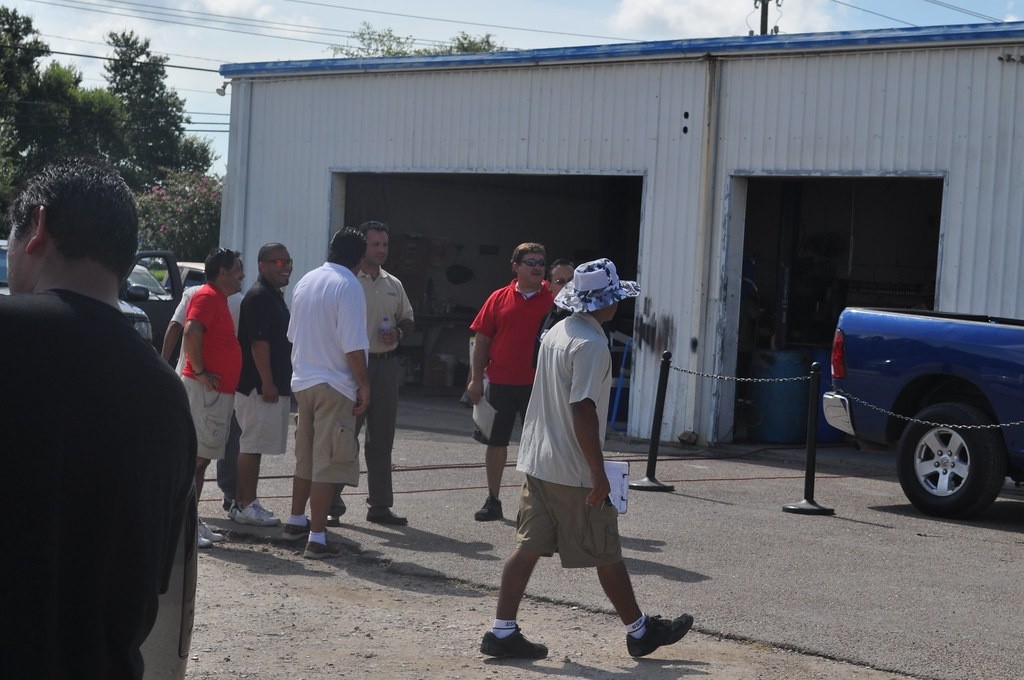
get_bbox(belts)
[367,348,400,360]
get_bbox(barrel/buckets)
[746,344,848,444]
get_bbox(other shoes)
[304,542,342,559]
[196,522,224,546]
[366,507,408,525]
[223,498,231,511]
[326,514,339,526]
[282,517,329,541]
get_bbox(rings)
[362,403,364,405]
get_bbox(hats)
[553,258,641,312]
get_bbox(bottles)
[380,316,392,335]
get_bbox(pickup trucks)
[821,304,1024,523]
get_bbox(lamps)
[215,81,230,97]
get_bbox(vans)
[162,262,206,296]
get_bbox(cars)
[117,249,188,373]
[0,240,16,299]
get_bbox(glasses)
[551,278,574,286]
[263,258,293,270]
[519,259,546,267]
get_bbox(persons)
[466,243,576,521]
[480,258,693,659]
[0,157,198,680]
[161,222,416,558]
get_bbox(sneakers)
[627,613,694,657]
[474,497,503,521]
[480,624,548,660]
[228,498,282,526]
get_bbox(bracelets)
[397,327,403,341]
[192,368,205,377]
[471,375,484,384]
